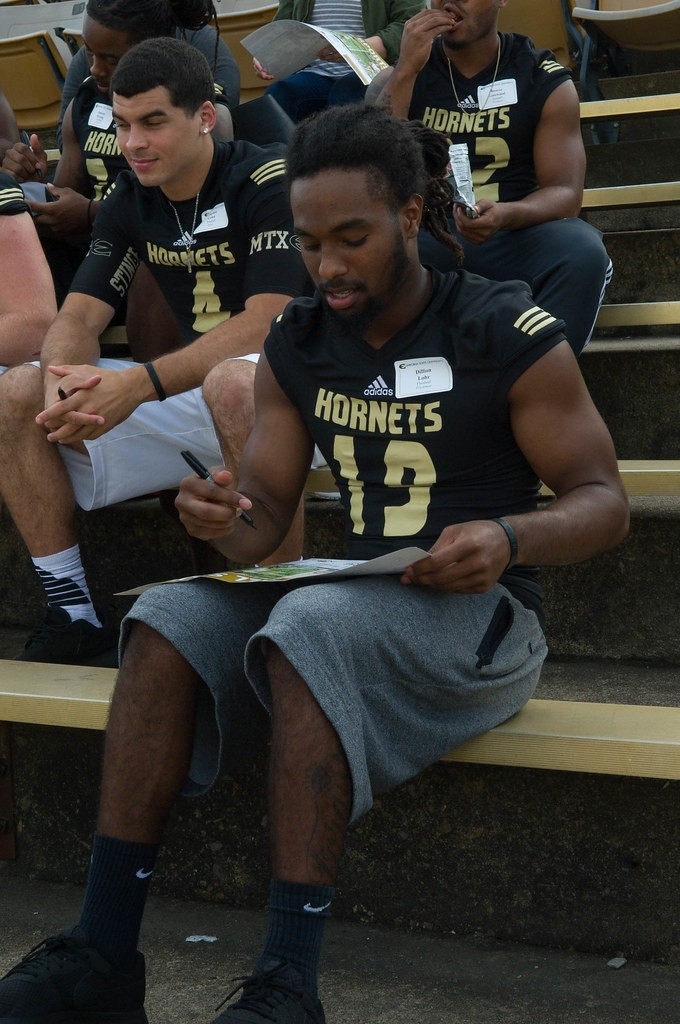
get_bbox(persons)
[252,0,429,129]
[0,0,227,365]
[56,17,240,218]
[0,104,629,1024]
[305,0,613,501]
[0,169,58,376]
[0,89,21,166]
[0,37,330,668]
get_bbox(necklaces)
[446,32,501,117]
[167,191,202,273]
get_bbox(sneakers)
[0,934,149,1024]
[209,968,324,1024]
[15,605,120,668]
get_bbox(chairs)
[1,0,680,143]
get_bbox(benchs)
[0,94,680,779]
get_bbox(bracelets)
[87,198,96,229]
[144,361,166,402]
[491,517,518,574]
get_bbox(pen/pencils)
[21,130,42,178]
[180,450,256,529]
[58,386,67,400]
